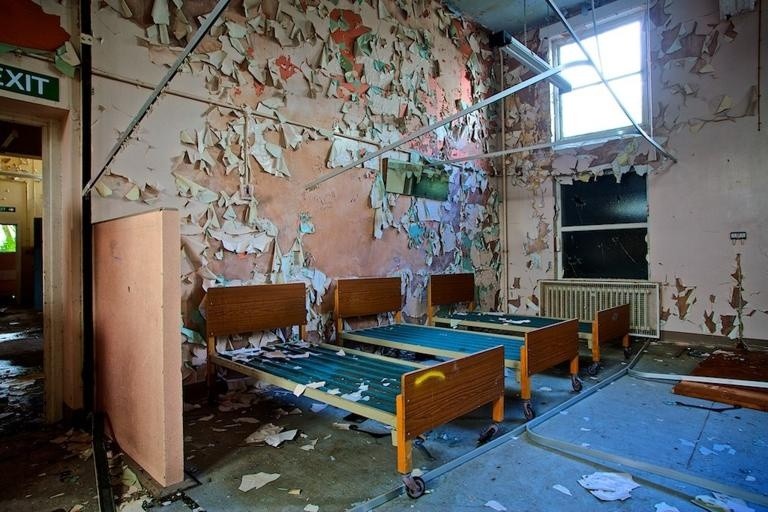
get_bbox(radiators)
[540,279,659,339]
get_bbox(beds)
[207,282,504,499]
[428,273,630,375]
[335,276,581,421]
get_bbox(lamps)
[492,0,572,90]
